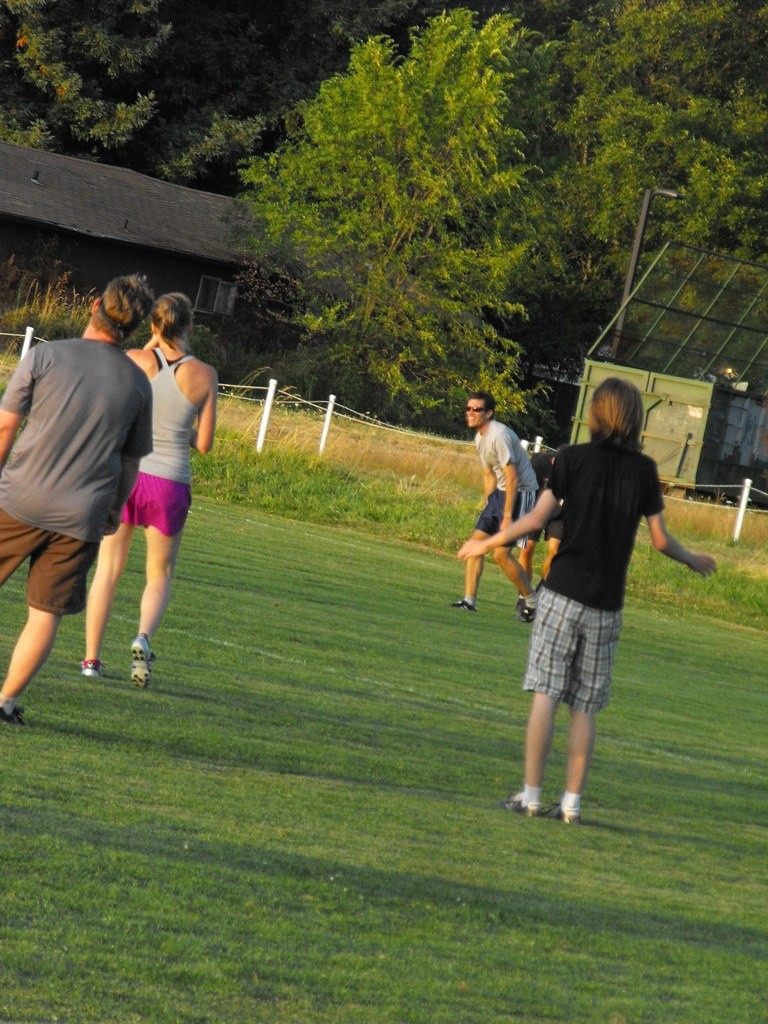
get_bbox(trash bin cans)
[570,357,768,504]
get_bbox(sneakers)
[131,635,156,689]
[81,659,101,676]
[0,704,25,727]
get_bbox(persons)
[448,390,541,623]
[0,274,153,726]
[457,378,719,824]
[515,443,570,623]
[80,293,219,689]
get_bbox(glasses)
[465,406,487,412]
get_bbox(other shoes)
[500,792,540,818]
[539,803,581,824]
[452,600,476,612]
[516,590,526,610]
[519,605,538,622]
